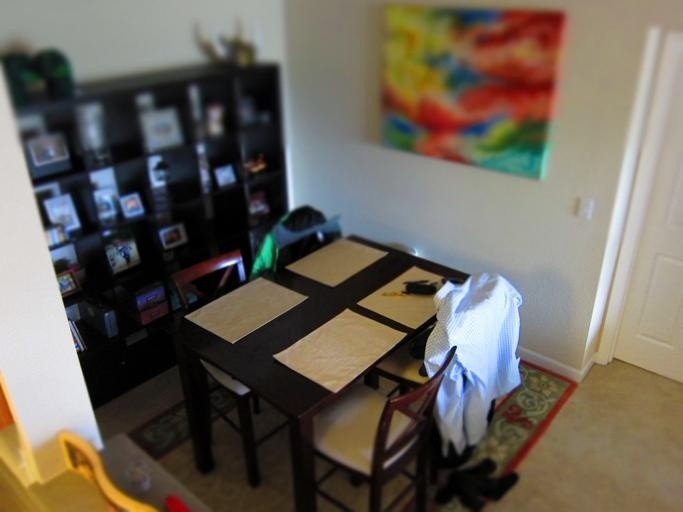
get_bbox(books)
[67,319,88,353]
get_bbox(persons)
[113,238,134,266]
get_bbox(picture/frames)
[20,109,71,169]
[32,179,86,248]
[159,222,190,253]
[136,92,186,153]
[87,166,147,223]
[104,236,144,276]
[75,100,132,154]
[194,141,239,194]
[54,268,83,301]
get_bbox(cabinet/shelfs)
[12,60,290,409]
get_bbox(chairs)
[172,248,287,490]
[312,342,459,512]
[276,228,345,271]
[362,327,459,395]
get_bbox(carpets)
[122,356,579,512]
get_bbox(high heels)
[432,457,520,512]
